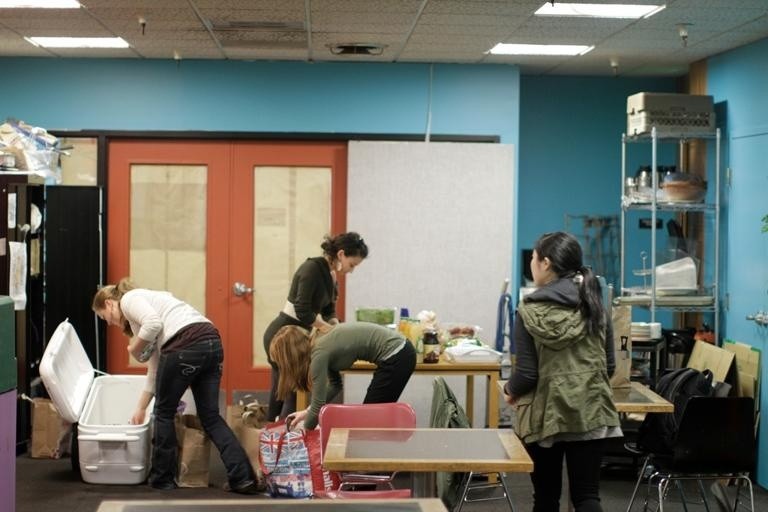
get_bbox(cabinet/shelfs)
[621,127,723,347]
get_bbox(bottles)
[399,317,407,335]
[423,332,440,363]
[410,319,424,364]
[405,318,412,339]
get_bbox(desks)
[603,381,675,416]
[297,327,500,488]
[322,428,533,512]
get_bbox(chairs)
[641,398,754,512]
[435,376,516,512]
[318,403,416,500]
[623,381,732,511]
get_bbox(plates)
[630,322,651,345]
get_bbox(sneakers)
[222,481,255,494]
[146,477,175,490]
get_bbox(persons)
[264,231,369,423]
[502,231,616,512]
[91,278,259,494]
[268,320,417,491]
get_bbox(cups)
[651,322,663,343]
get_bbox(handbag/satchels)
[255,419,338,498]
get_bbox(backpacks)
[636,367,716,461]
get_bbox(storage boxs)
[42,318,152,486]
[626,91,716,136]
